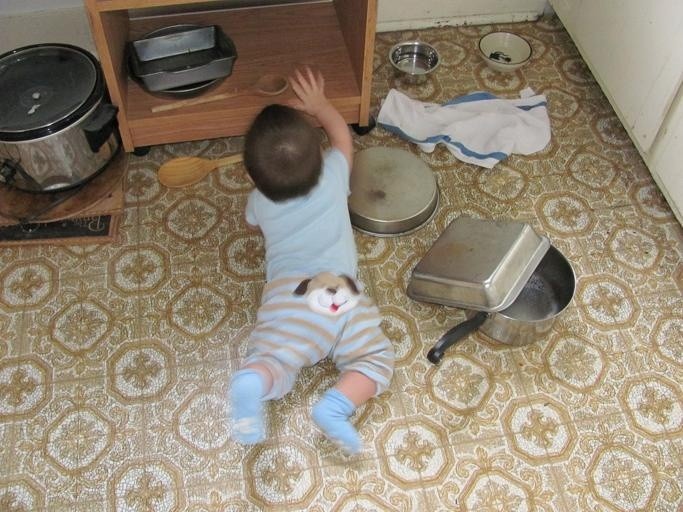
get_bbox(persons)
[226,64,395,454]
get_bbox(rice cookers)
[0,42,123,194]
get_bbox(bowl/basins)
[478,31,532,71]
[389,43,439,84]
[350,146,439,237]
[126,24,236,98]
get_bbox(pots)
[430,244,575,362]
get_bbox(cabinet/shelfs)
[84,0,383,155]
